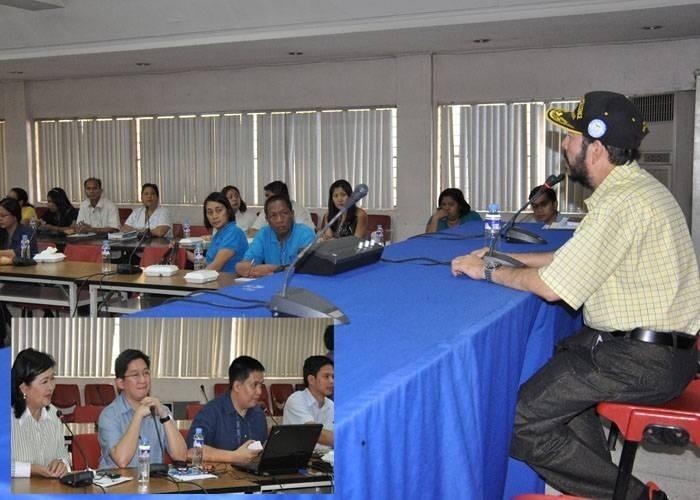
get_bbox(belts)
[630,328,698,350]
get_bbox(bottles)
[484,204,501,251]
[193,242,203,270]
[138,438,150,482]
[20,235,30,261]
[192,428,204,475]
[101,240,112,272]
[375,232,382,246]
[183,216,190,238]
[30,216,40,241]
[375,225,384,247]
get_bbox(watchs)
[484,261,503,282]
[159,413,172,424]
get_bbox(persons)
[183,354,268,464]
[424,188,483,235]
[235,193,317,277]
[444,90,700,500]
[171,191,250,275]
[0,177,123,348]
[10,348,74,480]
[93,347,188,468]
[282,325,334,451]
[515,183,569,224]
[198,184,259,242]
[248,180,316,239]
[119,182,175,238]
[316,178,368,239]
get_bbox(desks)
[74,267,261,318]
[0,261,139,317]
[1,221,585,499]
[36,233,253,253]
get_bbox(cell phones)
[97,469,121,478]
[174,460,188,471]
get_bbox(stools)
[597,375,700,500]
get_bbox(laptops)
[231,423,324,475]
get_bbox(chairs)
[311,213,318,227]
[119,208,132,225]
[64,243,103,263]
[130,247,186,298]
[36,207,48,220]
[367,214,391,240]
[74,208,79,214]
[51,384,309,471]
[37,242,56,252]
[172,224,183,239]
[190,226,213,237]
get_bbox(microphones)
[94,384,109,407]
[13,221,41,266]
[478,173,558,269]
[200,384,210,404]
[270,185,370,328]
[500,173,565,246]
[56,409,94,488]
[260,402,278,425]
[149,405,169,477]
[117,228,149,275]
[138,206,151,242]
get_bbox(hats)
[545,91,650,151]
